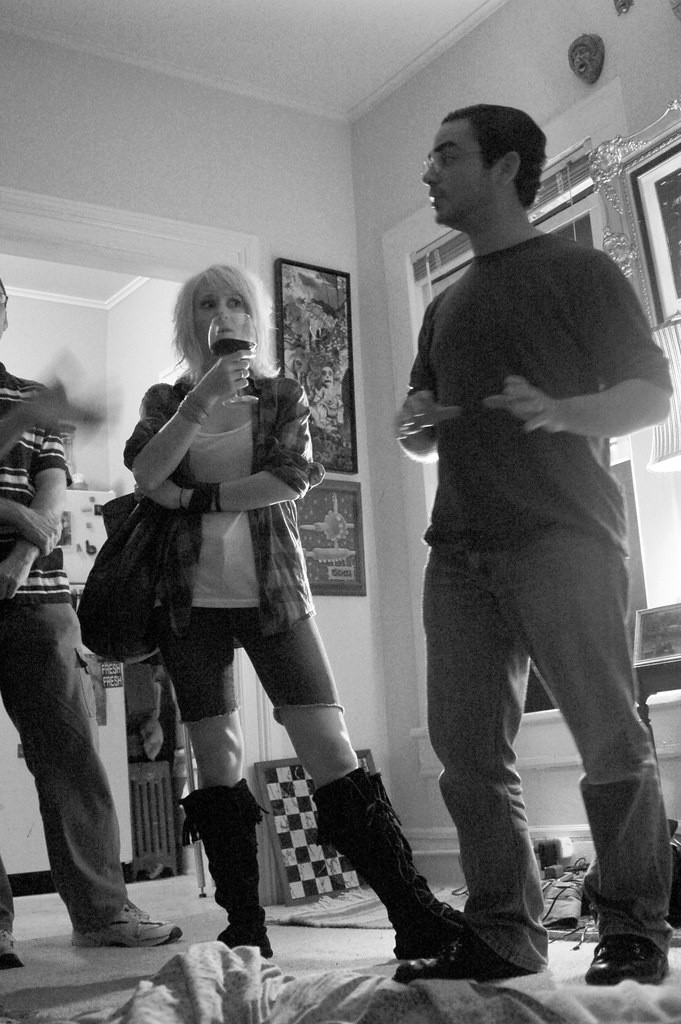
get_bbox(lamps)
[647,311,681,473]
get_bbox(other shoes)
[392,928,531,985]
[586,933,669,986]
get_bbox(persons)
[124,263,467,961]
[0,278,183,968]
[0,390,108,464]
[392,102,673,985]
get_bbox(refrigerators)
[0,489,132,887]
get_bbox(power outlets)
[534,837,574,858]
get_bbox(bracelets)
[179,488,185,512]
[177,391,211,426]
[187,483,222,514]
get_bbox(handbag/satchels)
[76,382,193,663]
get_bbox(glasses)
[421,150,483,175]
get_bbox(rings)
[241,370,243,379]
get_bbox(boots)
[178,778,273,959]
[312,767,466,961]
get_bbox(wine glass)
[208,314,259,407]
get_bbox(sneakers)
[1,929,23,967]
[72,900,182,947]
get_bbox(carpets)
[263,883,681,948]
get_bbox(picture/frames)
[588,99,681,328]
[273,258,359,476]
[632,603,681,668]
[253,749,376,907]
[294,480,367,598]
